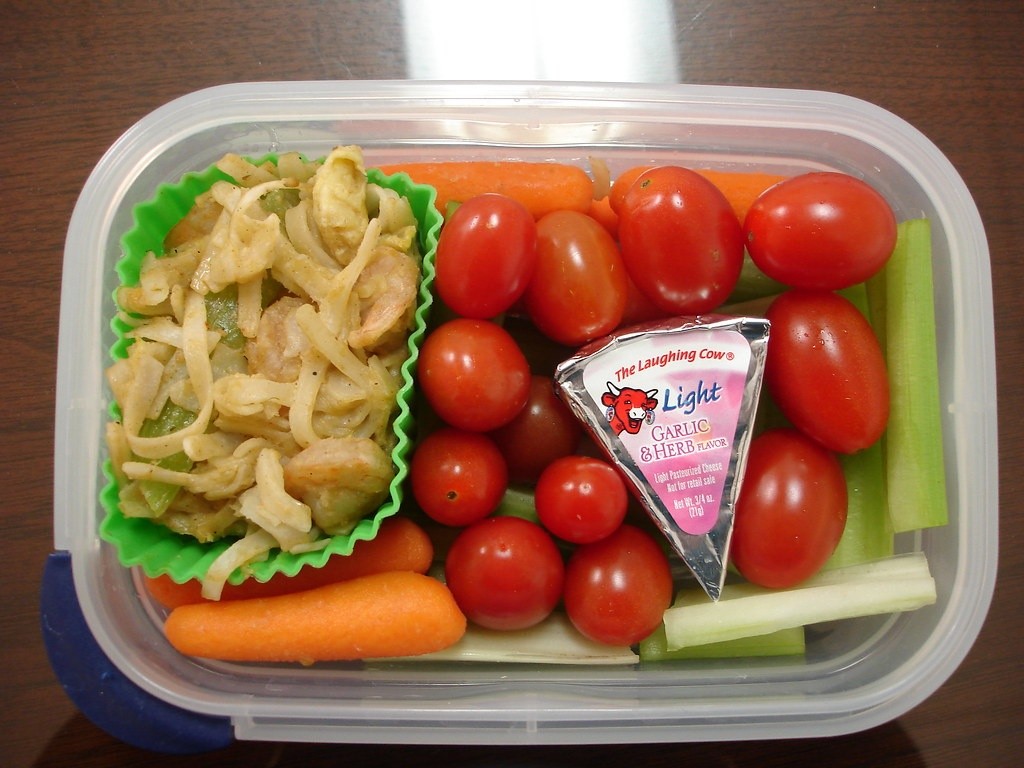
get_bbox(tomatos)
[407,165,898,648]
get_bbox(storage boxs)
[43,78,1002,751]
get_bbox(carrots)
[138,522,467,666]
[373,160,792,236]
